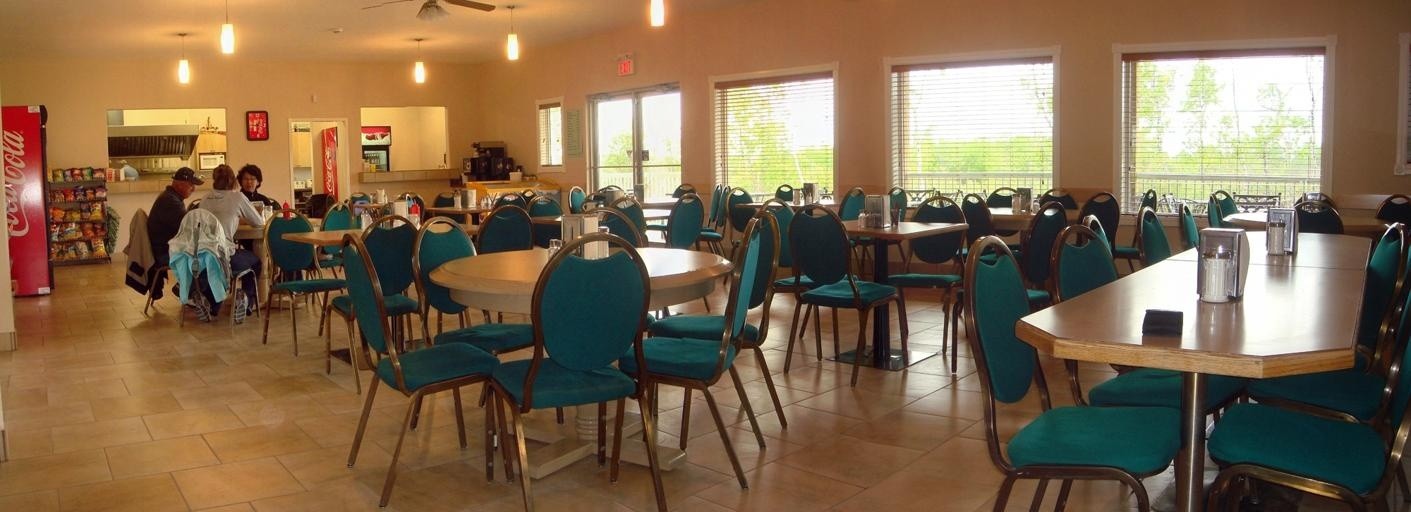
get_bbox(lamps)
[221,0,234,54]
[178,33,189,84]
[414,39,425,83]
[416,2,449,20]
[650,1,665,28]
[506,6,518,59]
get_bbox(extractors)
[108,124,199,161]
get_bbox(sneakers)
[232,288,248,324]
[191,290,213,323]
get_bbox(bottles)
[598,225,609,259]
[548,239,562,258]
[1033,198,1040,214]
[361,210,373,230]
[891,208,901,224]
[793,188,801,204]
[264,206,273,221]
[409,201,420,224]
[454,190,462,209]
[1012,193,1022,215]
[599,200,605,211]
[283,200,290,219]
[805,195,813,205]
[364,152,381,164]
[1268,222,1285,256]
[1200,250,1230,303]
[858,212,867,229]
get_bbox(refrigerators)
[3,104,55,297]
[321,125,391,202]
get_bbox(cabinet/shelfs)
[47,178,108,265]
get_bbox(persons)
[148,168,204,296]
[120,159,139,178]
[235,164,277,210]
[192,163,261,325]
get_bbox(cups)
[510,172,522,181]
[377,190,385,203]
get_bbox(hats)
[171,167,205,185]
[119,160,128,165]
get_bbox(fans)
[361,0,496,12]
